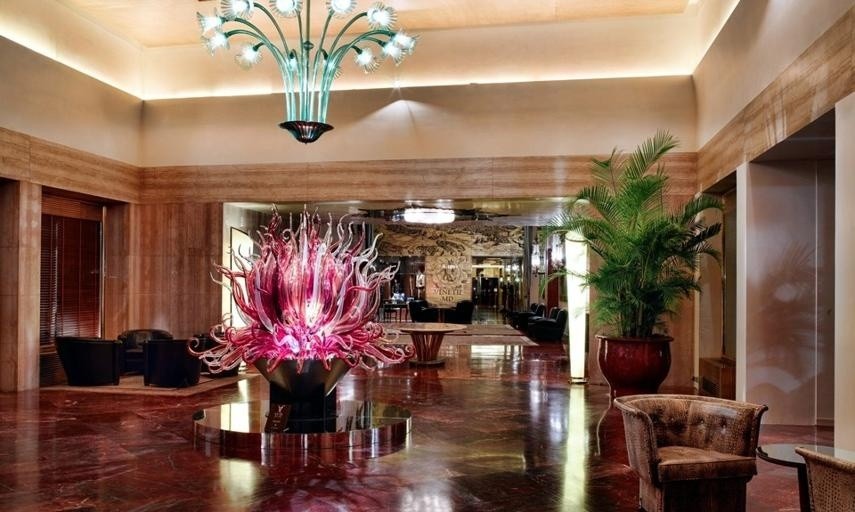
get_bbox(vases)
[192,357,409,447]
[594,399,629,463]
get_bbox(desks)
[377,304,408,323]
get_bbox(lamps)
[196,0,421,145]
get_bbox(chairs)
[795,446,855,512]
[193,332,240,378]
[54,336,122,386]
[409,300,474,324]
[142,338,204,388]
[506,303,568,343]
[118,328,173,375]
[383,299,398,322]
[613,393,768,512]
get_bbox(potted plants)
[536,131,726,407]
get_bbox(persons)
[393,288,401,304]
[399,292,407,304]
[508,284,515,313]
[391,279,402,295]
[415,268,426,300]
[499,281,507,311]
[473,287,478,313]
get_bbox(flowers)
[187,204,416,373]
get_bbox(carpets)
[381,335,538,346]
[39,372,261,396]
[376,322,524,335]
